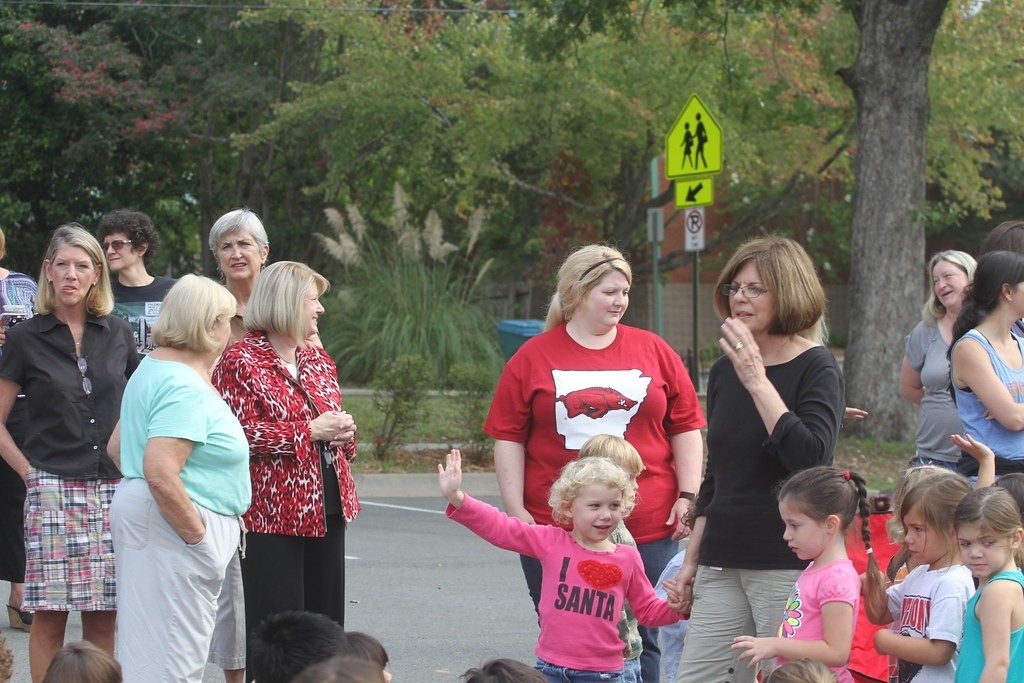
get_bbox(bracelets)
[679,492,698,502]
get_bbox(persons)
[483,245,709,683]
[435,434,689,683]
[668,235,845,683]
[248,609,392,683]
[0,224,142,683]
[211,261,362,624]
[43,639,122,683]
[105,274,252,683]
[96,209,177,362]
[209,207,269,374]
[730,435,1024,683]
[899,220,1024,490]
[0,228,39,633]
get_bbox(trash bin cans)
[499,319,544,365]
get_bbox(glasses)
[104,239,131,251]
[78,357,92,395]
[719,283,768,298]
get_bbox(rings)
[735,342,743,350]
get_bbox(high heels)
[7,605,33,632]
